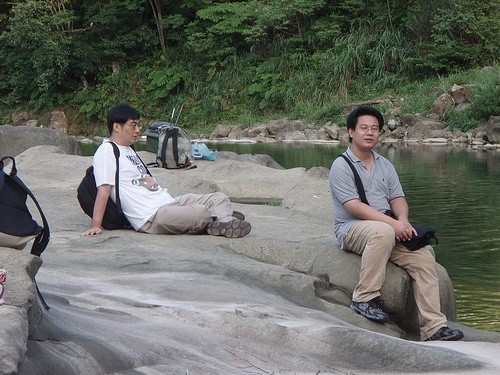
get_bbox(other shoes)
[206,218,251,238]
[212,211,245,220]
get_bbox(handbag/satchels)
[385,208,435,251]
[192,143,211,159]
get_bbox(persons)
[329,106,464,342]
[81,102,252,239]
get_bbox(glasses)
[354,125,380,133]
[117,121,143,129]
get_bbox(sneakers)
[426,327,464,341]
[352,299,389,322]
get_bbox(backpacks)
[76,141,130,230]
[156,126,192,168]
[0,155,50,257]
